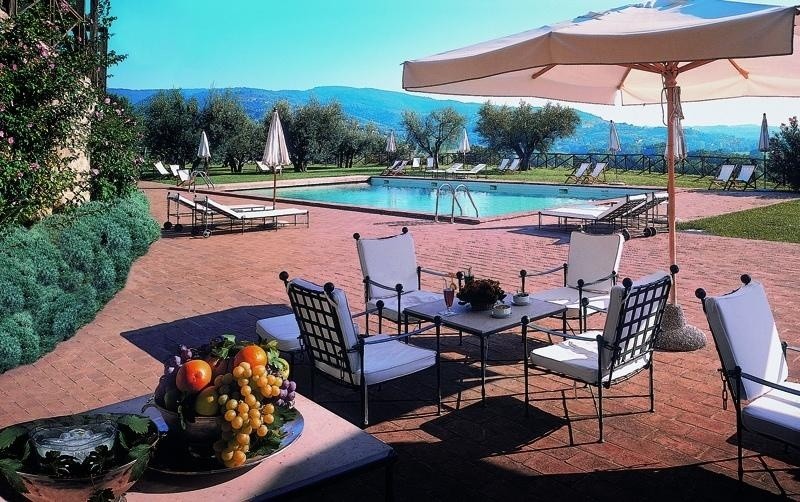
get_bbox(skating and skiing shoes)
[157,342,196,408]
[214,361,297,468]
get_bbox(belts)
[443,284,455,314]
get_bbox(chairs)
[565,162,593,183]
[380,160,409,175]
[410,157,488,179]
[729,163,759,191]
[696,275,800,502]
[282,270,442,426]
[709,164,737,190]
[353,228,463,347]
[153,160,196,192]
[521,266,676,444]
[519,229,625,341]
[255,159,282,175]
[492,157,522,174]
[581,161,610,185]
[164,190,276,233]
[193,194,310,238]
[537,193,668,227]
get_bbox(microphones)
[257,313,301,356]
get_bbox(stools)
[257,313,301,356]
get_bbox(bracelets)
[2,394,227,502]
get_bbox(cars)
[464,271,474,284]
[492,304,511,318]
[512,294,530,305]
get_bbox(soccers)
[163,390,185,409]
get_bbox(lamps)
[492,157,522,174]
[255,159,282,175]
[729,163,759,191]
[193,194,310,238]
[709,164,737,190]
[565,162,593,183]
[581,161,610,185]
[164,190,276,233]
[153,160,196,192]
[410,157,488,179]
[380,160,409,175]
[537,193,668,227]
[696,275,800,502]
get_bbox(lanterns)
[194,386,220,416]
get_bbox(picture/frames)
[0,392,394,502]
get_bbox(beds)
[664,116,687,161]
[402,1,800,303]
[386,130,397,168]
[197,130,213,160]
[261,108,291,220]
[757,113,771,188]
[459,126,472,167]
[610,119,621,180]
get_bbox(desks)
[0,392,394,502]
[402,293,568,411]
